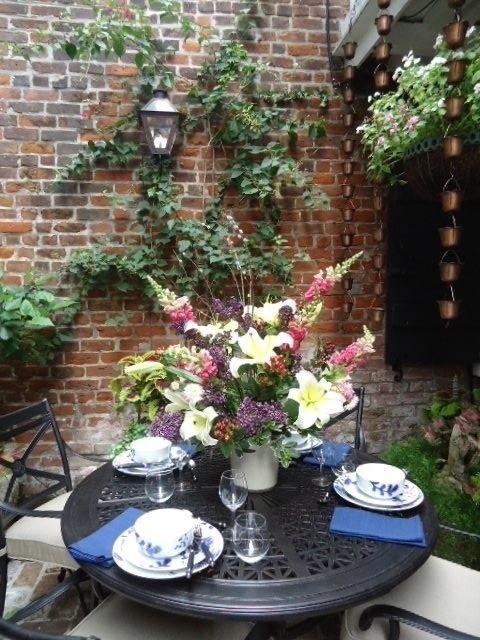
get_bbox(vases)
[230,444,279,490]
[399,133,479,195]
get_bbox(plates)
[121,526,211,570]
[112,518,224,580]
[285,432,323,451]
[344,475,420,507]
[113,446,188,477]
[331,472,424,512]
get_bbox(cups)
[232,514,269,565]
[144,467,175,503]
[356,462,405,499]
[136,507,195,557]
[129,437,171,461]
[330,452,360,477]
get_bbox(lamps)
[138,89,182,156]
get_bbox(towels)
[176,441,200,458]
[329,506,428,549]
[67,506,143,567]
[303,441,352,465]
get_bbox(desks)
[60,440,438,640]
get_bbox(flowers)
[351,23,479,191]
[421,404,480,498]
[108,210,376,467]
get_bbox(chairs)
[0,395,106,639]
[0,568,256,640]
[337,524,480,640]
[352,386,370,452]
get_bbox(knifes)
[117,463,162,468]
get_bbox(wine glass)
[311,430,334,488]
[219,470,248,541]
[169,438,193,492]
